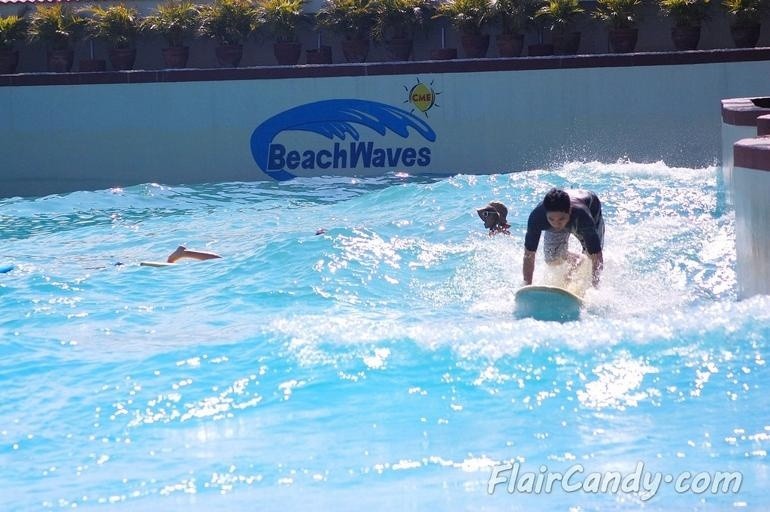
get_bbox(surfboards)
[514,257,592,303]
[141,261,179,266]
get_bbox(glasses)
[482,211,497,216]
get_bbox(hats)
[478,202,507,223]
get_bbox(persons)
[167,246,222,264]
[523,188,605,293]
[477,202,512,236]
